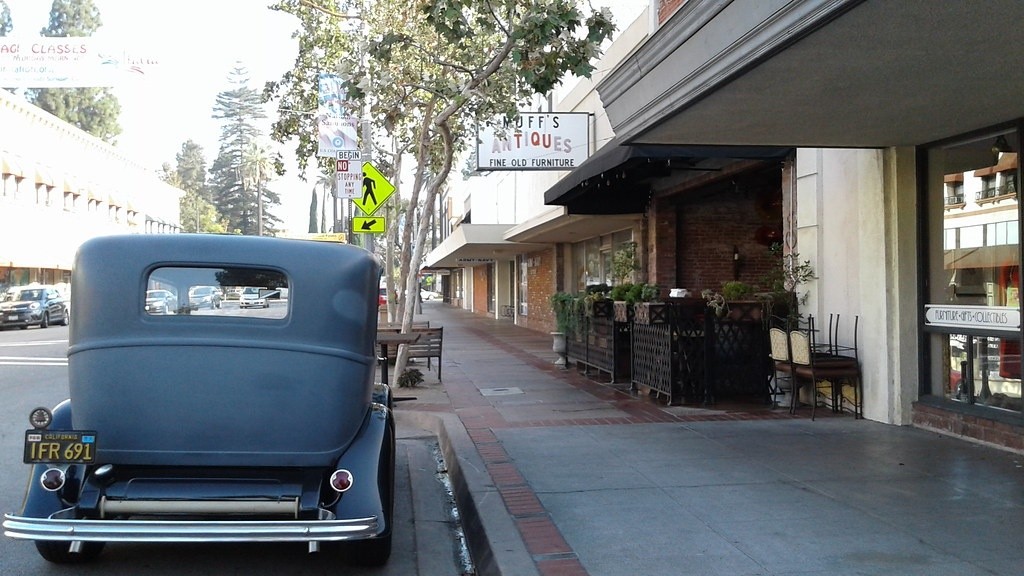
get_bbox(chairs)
[767,314,862,422]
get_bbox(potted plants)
[548,292,573,365]
[611,283,666,325]
[584,283,612,317]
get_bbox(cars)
[396,286,439,301]
[378,287,399,305]
[239,286,269,309]
[0,283,70,330]
[1,230,396,570]
[187,285,223,312]
[949,367,963,400]
[216,287,242,301]
[145,289,177,315]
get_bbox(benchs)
[377,320,443,382]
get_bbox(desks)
[377,333,421,402]
